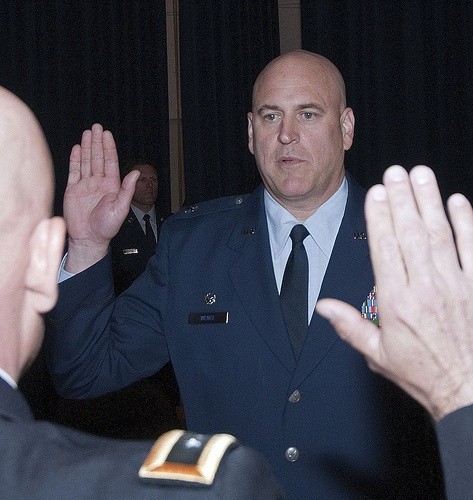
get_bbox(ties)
[143,214,156,259]
[280,224,310,364]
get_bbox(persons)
[110,160,166,299]
[46,49,446,500]
[0,83,473,500]
